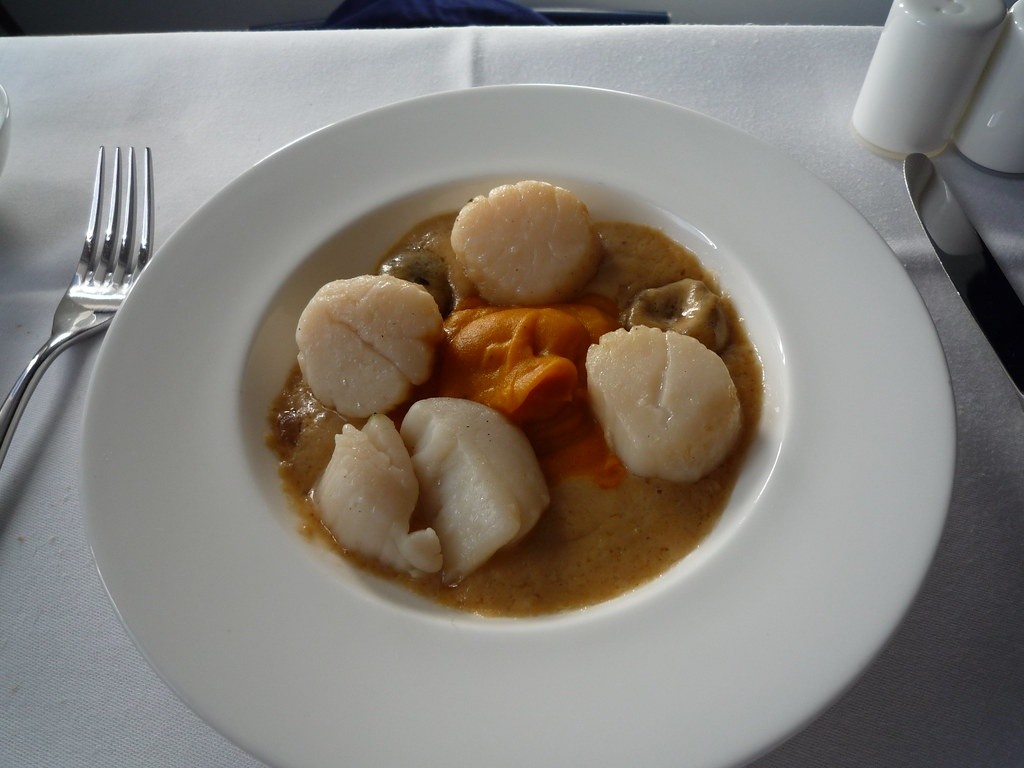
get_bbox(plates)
[79,83,957,768]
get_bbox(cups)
[853,0,1024,178]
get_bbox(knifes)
[903,153,1024,398]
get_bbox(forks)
[0,146,155,469]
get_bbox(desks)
[0,25,1024,768]
